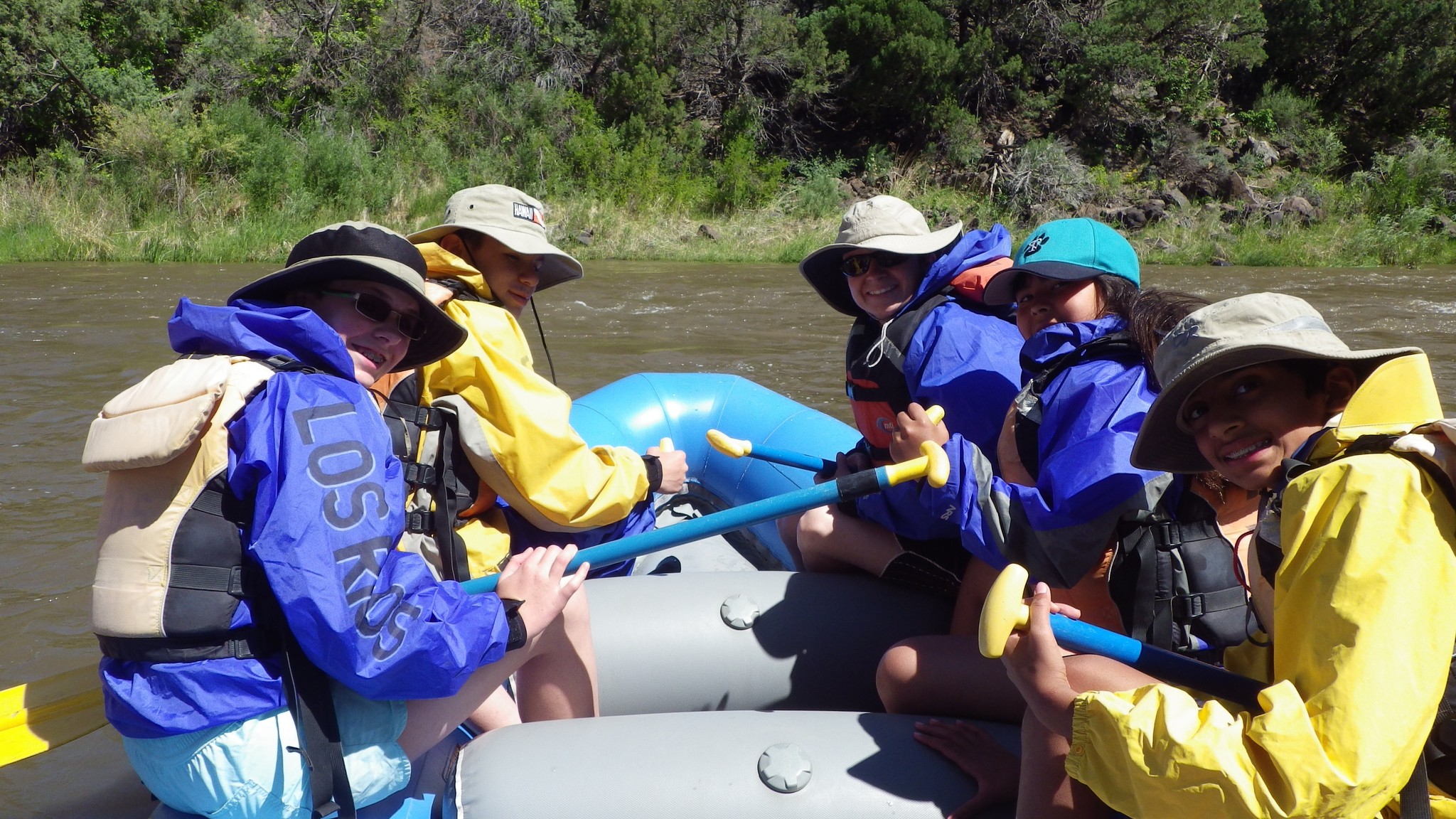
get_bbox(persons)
[775,196,1025,581]
[874,218,1172,715]
[81,218,602,819]
[368,184,689,578]
[915,293,1456,819]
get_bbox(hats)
[228,217,471,374]
[1128,292,1432,473]
[404,184,583,291]
[981,217,1141,306]
[798,194,968,317]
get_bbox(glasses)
[305,287,429,340]
[840,250,914,279]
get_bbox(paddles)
[706,426,838,477]
[973,559,1263,709]
[924,403,945,427]
[0,438,952,771]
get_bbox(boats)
[148,369,867,819]
